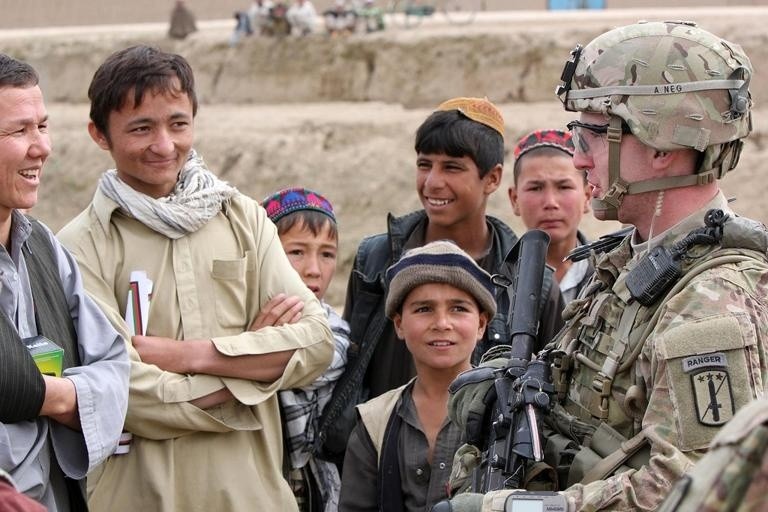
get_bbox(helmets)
[556,19,752,150]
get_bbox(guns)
[473,231,557,493]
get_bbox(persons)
[232,2,461,43]
[1,58,133,509]
[53,44,337,511]
[415,16,768,511]
[657,389,768,512]
[317,93,567,509]
[507,130,598,312]
[255,183,354,510]
[337,237,509,509]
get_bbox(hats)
[514,129,587,171]
[260,188,338,223]
[433,98,504,140]
[384,238,497,323]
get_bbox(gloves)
[446,344,515,449]
[430,489,483,511]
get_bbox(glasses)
[566,120,631,152]
